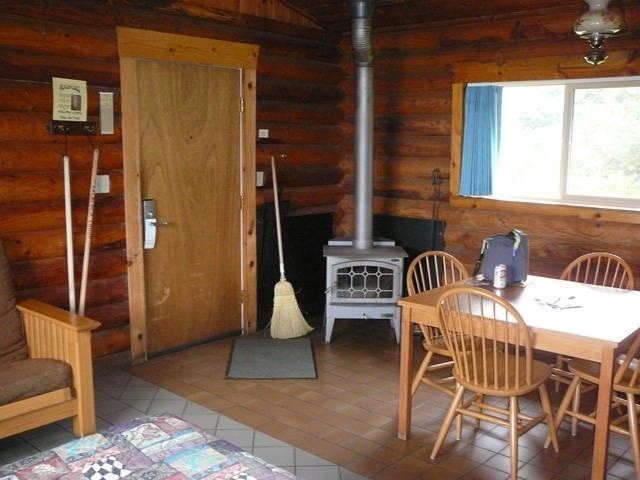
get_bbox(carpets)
[1,411,297,479]
[223,334,319,380]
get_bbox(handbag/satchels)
[464,229,529,288]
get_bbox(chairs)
[405,250,522,443]
[550,249,636,437]
[1,249,103,438]
[429,285,561,480]
[544,327,640,480]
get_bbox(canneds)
[493,264,508,289]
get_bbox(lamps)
[573,0,631,66]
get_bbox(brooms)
[267,155,314,341]
